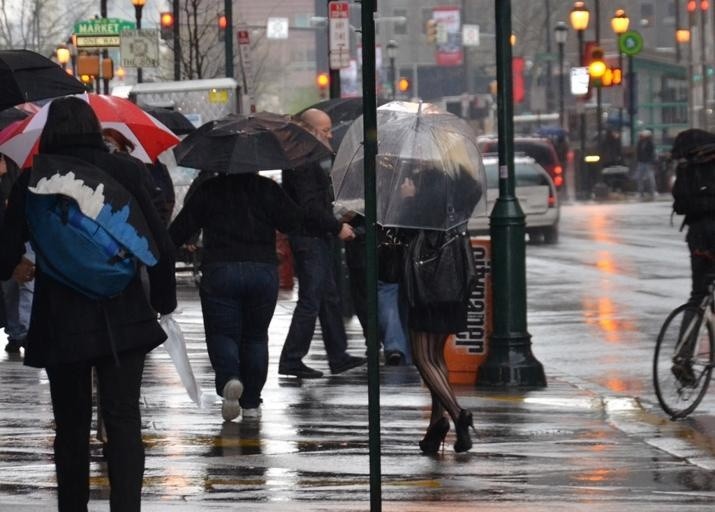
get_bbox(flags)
[433,6,466,68]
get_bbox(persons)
[536,112,677,200]
[668,128,715,387]
[0,98,486,510]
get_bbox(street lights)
[611,9,629,69]
[386,40,398,103]
[56,0,146,95]
[570,1,590,150]
[555,22,569,126]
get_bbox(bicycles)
[653,250,715,419]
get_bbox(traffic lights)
[399,77,411,92]
[317,73,330,87]
[426,19,440,45]
[586,42,622,86]
[161,13,175,39]
[218,15,226,41]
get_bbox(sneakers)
[386,348,402,366]
[6,338,28,353]
[279,362,323,378]
[222,379,243,421]
[329,355,366,373]
[241,405,263,418]
[672,358,697,387]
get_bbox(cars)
[467,152,558,244]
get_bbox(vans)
[476,135,563,193]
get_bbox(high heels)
[419,415,450,452]
[453,411,480,451]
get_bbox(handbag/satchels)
[402,212,478,309]
[25,169,163,302]
[682,180,715,224]
[379,240,402,283]
